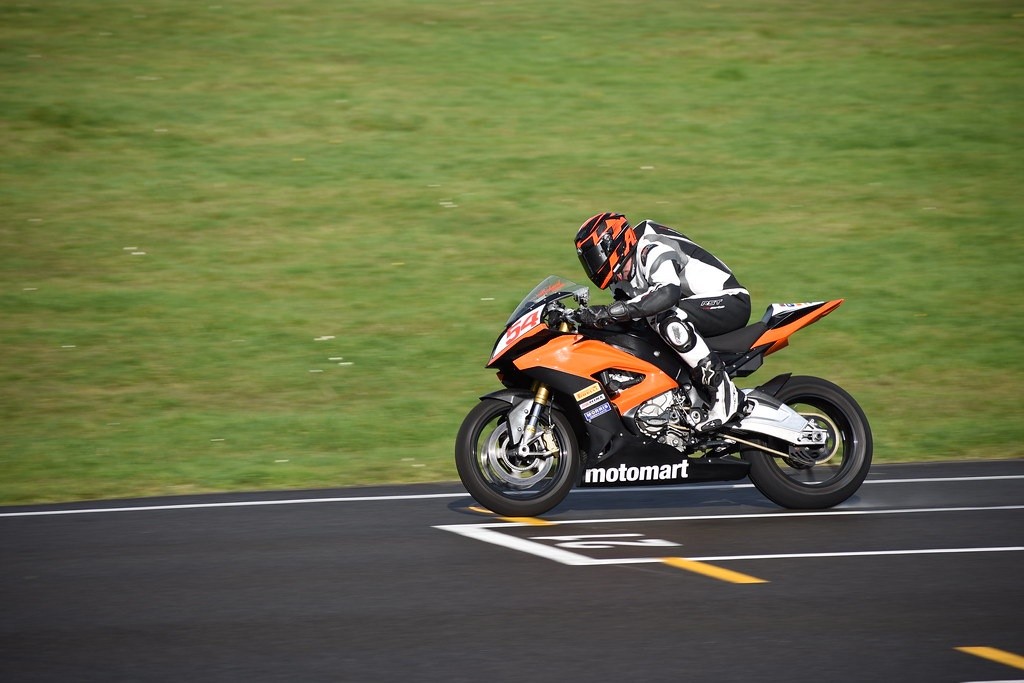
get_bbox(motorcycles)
[455,273,873,518]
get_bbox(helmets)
[574,211,637,290]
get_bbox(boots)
[691,351,746,432]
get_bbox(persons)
[575,213,751,433]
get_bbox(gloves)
[579,299,629,328]
